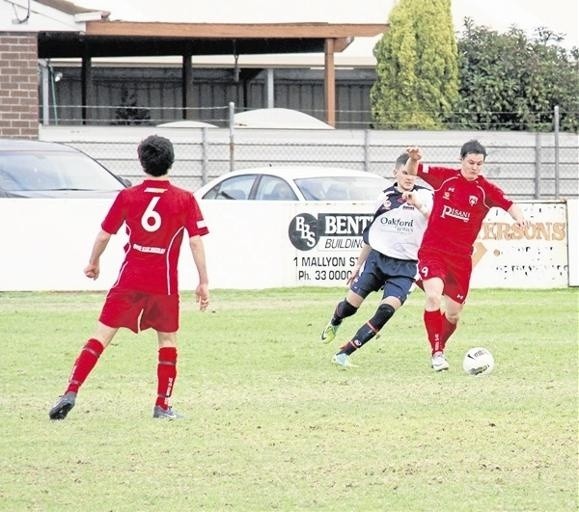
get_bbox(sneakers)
[320,316,341,345]
[330,350,352,375]
[429,352,450,373]
[153,405,185,421]
[47,391,76,421]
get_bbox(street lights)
[48,61,63,125]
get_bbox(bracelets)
[419,204,427,215]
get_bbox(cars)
[0,137,138,202]
[191,160,397,202]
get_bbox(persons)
[48,133,211,418]
[321,153,435,368]
[406,140,531,372]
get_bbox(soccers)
[463,348,493,377]
